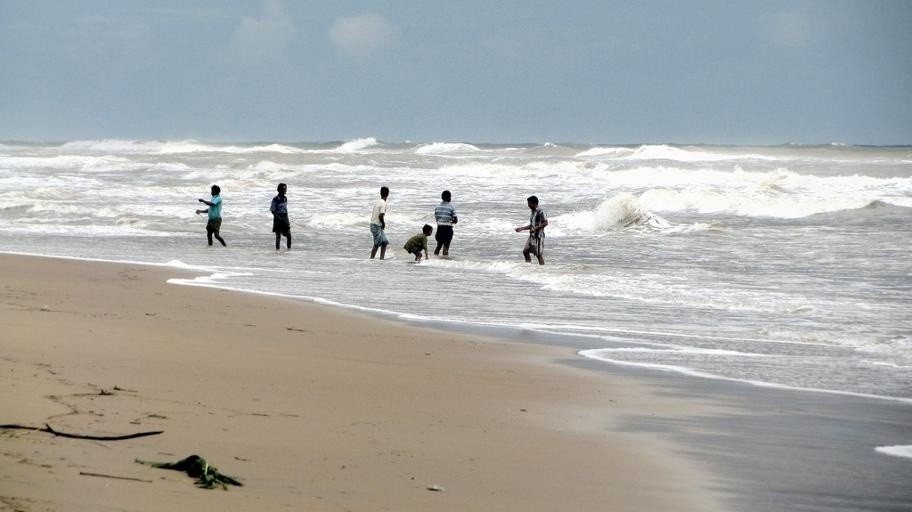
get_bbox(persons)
[514,195,549,265]
[434,190,458,257]
[367,186,390,260]
[269,182,292,250]
[402,223,433,262]
[194,184,227,247]
